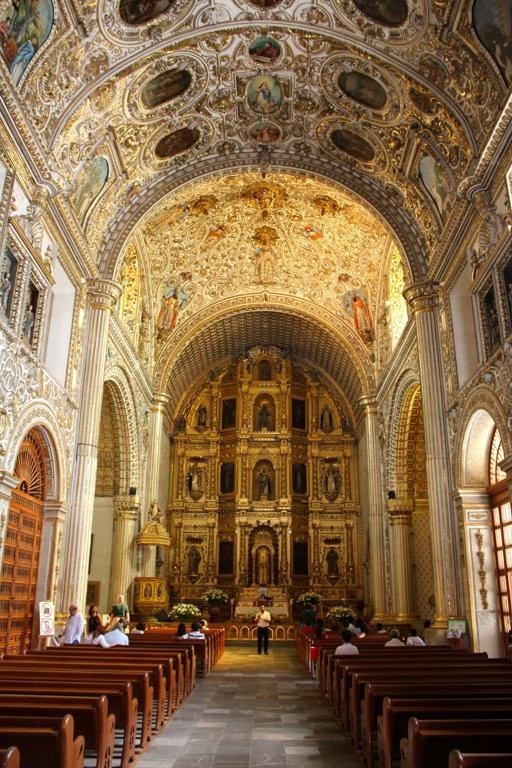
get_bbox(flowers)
[202,587,229,607]
[296,591,323,607]
[325,604,357,623]
[168,601,200,621]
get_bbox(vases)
[302,609,313,618]
[208,605,220,616]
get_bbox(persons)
[131,623,148,634]
[58,604,83,645]
[86,604,129,649]
[200,619,209,630]
[174,623,188,638]
[187,544,201,574]
[335,629,359,654]
[385,628,405,646]
[405,628,426,647]
[322,460,338,492]
[109,594,129,623]
[255,603,272,654]
[320,404,332,432]
[257,468,271,499]
[190,466,200,491]
[172,622,206,639]
[258,402,269,428]
[297,615,387,638]
[325,547,339,576]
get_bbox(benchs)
[0,623,228,767]
[293,625,512,768]
